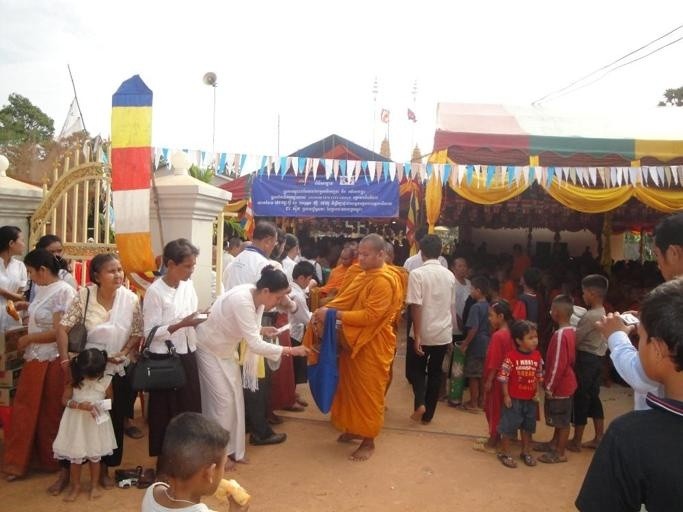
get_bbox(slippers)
[137,468,155,489]
[125,426,145,439]
[497,452,518,469]
[473,443,497,455]
[521,452,537,467]
[533,443,553,452]
[476,438,488,445]
[536,451,568,465]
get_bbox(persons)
[194,265,311,474]
[567,275,610,451]
[472,300,514,456]
[288,261,317,384]
[453,276,492,414]
[223,237,242,270]
[0,248,78,483]
[442,255,472,403]
[312,248,355,309]
[533,294,577,464]
[269,227,309,412]
[52,348,117,502]
[595,211,683,410]
[282,233,299,283]
[132,238,212,477]
[405,234,455,426]
[222,220,288,446]
[513,267,544,324]
[0,226,28,331]
[26,234,79,302]
[49,253,144,497]
[459,238,664,285]
[311,233,405,464]
[340,242,409,312]
[140,411,250,512]
[574,275,683,511]
[497,321,543,468]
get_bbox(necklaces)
[162,487,196,506]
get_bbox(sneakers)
[250,432,287,445]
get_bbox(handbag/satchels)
[130,326,188,391]
[114,466,143,489]
[66,286,92,353]
[306,309,340,414]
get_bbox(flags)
[381,109,390,123]
[408,109,417,122]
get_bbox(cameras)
[619,313,640,327]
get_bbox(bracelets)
[60,359,69,364]
[287,346,291,358]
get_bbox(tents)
[219,133,423,223]
[425,130,683,254]
